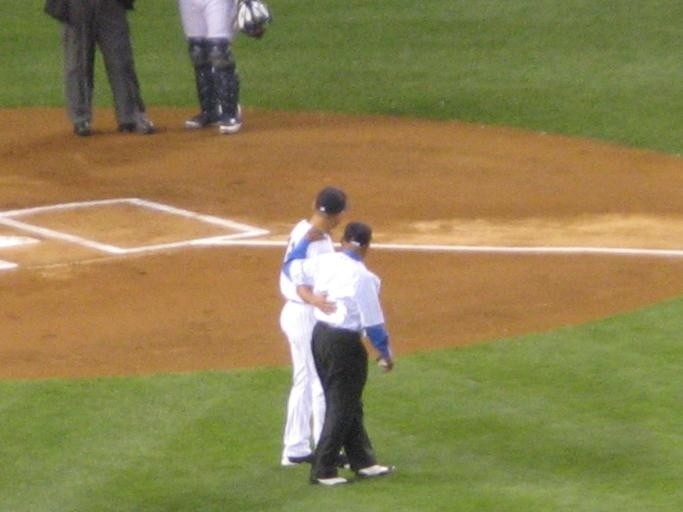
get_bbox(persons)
[282,221,395,486]
[179,0,271,133]
[43,0,156,135]
[279,187,351,467]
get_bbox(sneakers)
[74,120,91,135]
[280,452,315,466]
[118,118,154,134]
[335,451,352,469]
[184,113,222,128]
[310,476,352,486]
[219,117,242,134]
[356,464,395,479]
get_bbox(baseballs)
[377,359,388,370]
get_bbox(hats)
[315,187,347,214]
[344,221,372,247]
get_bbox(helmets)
[238,2,270,38]
